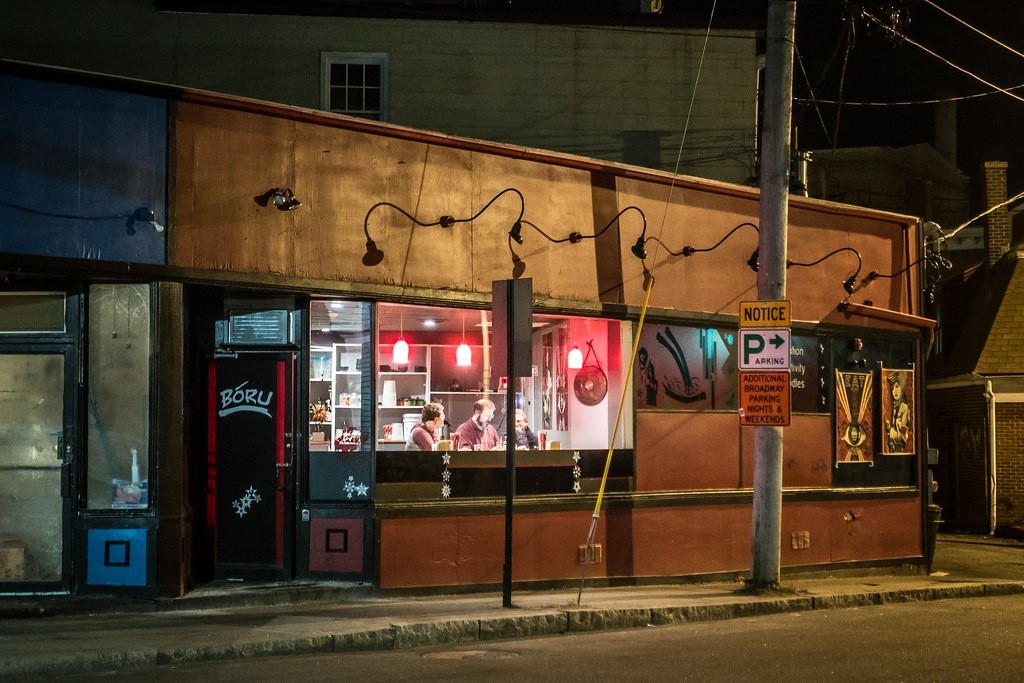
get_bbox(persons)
[455,399,501,450]
[515,409,539,449]
[405,403,445,451]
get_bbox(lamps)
[270,187,302,211]
[455,309,472,366]
[870,257,938,304]
[682,223,760,271]
[439,187,525,245]
[567,319,584,369]
[786,247,862,295]
[570,205,647,261]
[392,306,409,365]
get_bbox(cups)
[392,423,403,440]
[473,444,483,451]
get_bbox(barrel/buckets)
[403,414,421,441]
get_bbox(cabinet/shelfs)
[309,344,525,451]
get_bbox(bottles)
[540,432,546,450]
